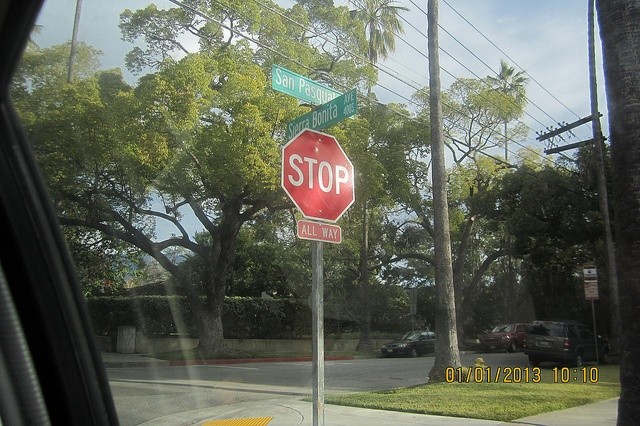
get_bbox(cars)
[381,330,435,357]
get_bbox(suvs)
[523,320,608,368]
[479,322,531,353]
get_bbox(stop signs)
[282,128,355,222]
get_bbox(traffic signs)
[296,219,341,243]
[285,87,358,145]
[272,62,356,119]
